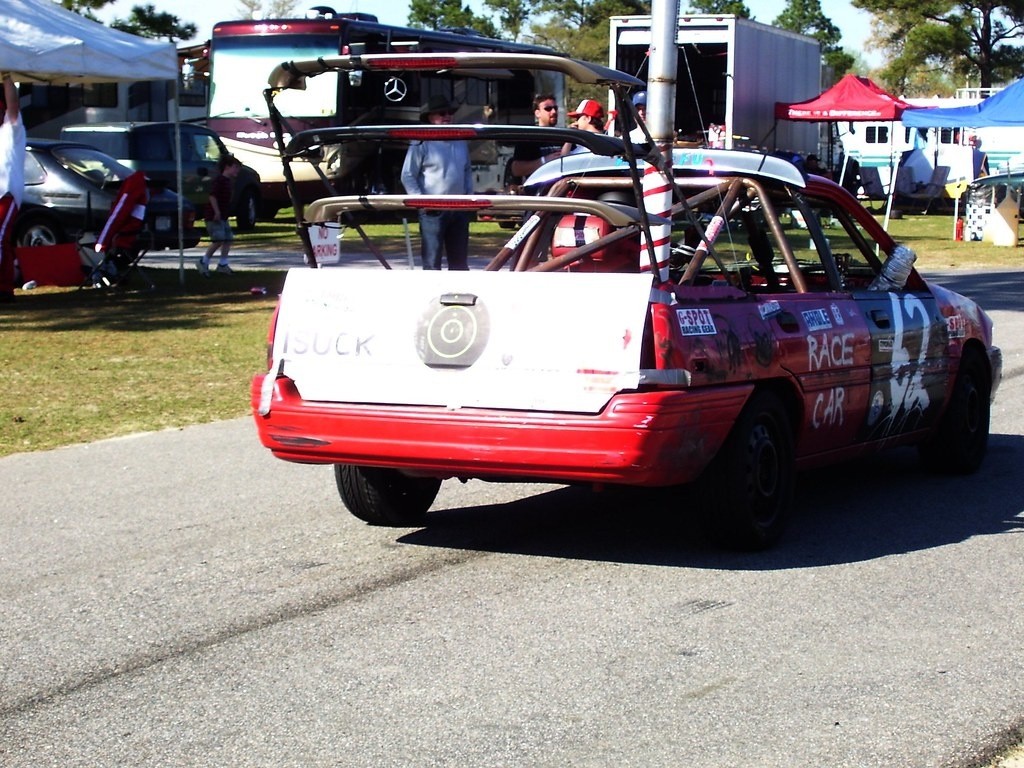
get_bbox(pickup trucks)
[250,145,1005,522]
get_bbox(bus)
[206,4,571,229]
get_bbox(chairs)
[72,171,155,298]
[861,165,951,217]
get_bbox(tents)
[882,77,1024,231]
[0,0,184,284]
[773,74,939,180]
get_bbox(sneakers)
[215,263,237,275]
[196,260,210,278]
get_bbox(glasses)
[540,105,558,112]
[436,110,455,116]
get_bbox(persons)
[401,90,474,271]
[804,154,832,179]
[195,155,239,276]
[616,91,678,144]
[973,139,990,179]
[0,71,27,303]
[560,99,604,156]
[511,95,577,261]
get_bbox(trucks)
[837,92,1024,194]
[607,13,860,229]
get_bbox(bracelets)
[541,156,546,164]
[2,73,10,77]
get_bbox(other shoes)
[0,292,16,303]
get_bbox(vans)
[60,122,263,227]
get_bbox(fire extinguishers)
[956,217,964,240]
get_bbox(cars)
[17,137,200,270]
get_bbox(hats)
[566,100,604,119]
[632,91,646,107]
[419,94,460,122]
[807,154,821,161]
